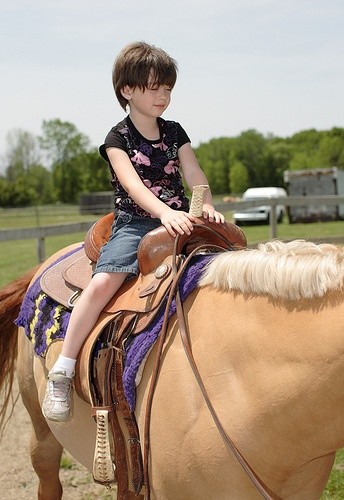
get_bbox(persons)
[41,42,225,423]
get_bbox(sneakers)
[41,371,75,422]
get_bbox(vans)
[233,187,288,224]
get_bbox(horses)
[1,238,344,500]
